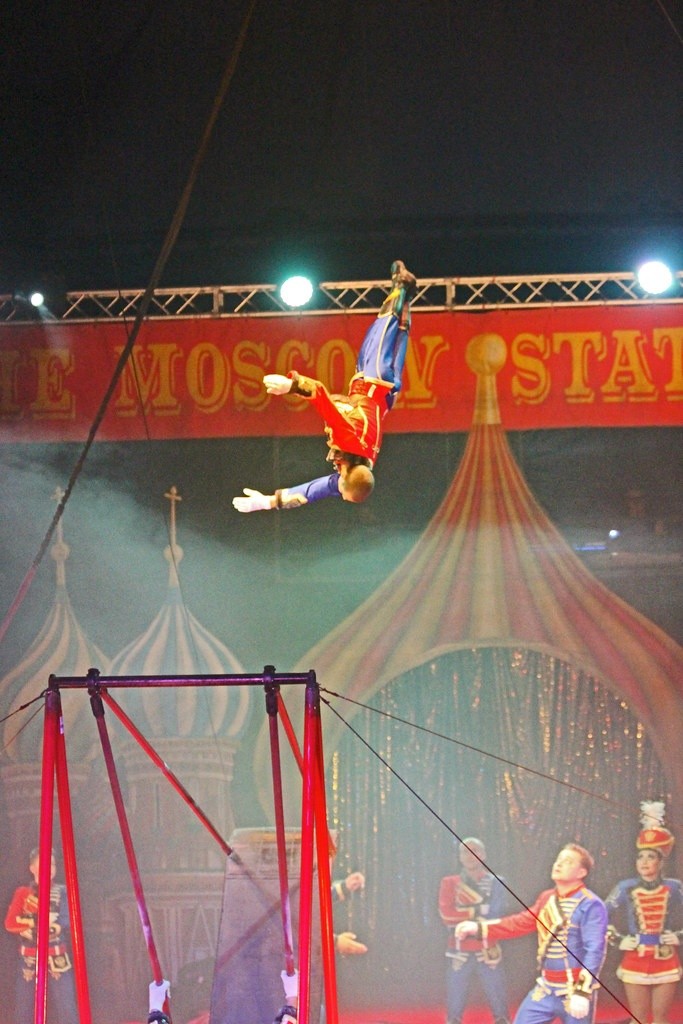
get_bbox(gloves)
[570,994,589,1019]
[281,968,298,998]
[455,920,478,941]
[149,978,171,1013]
[262,374,292,395]
[232,488,271,513]
[49,912,59,924]
[619,934,637,950]
[20,929,33,941]
[662,930,680,946]
[480,905,490,915]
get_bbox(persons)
[5,844,77,1023]
[330,869,369,957]
[602,799,682,1023]
[437,836,510,1024]
[148,968,302,1024]
[230,257,418,515]
[457,842,608,1023]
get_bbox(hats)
[637,800,675,857]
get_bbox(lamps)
[11,282,45,307]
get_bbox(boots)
[377,260,416,320]
[398,285,419,331]
